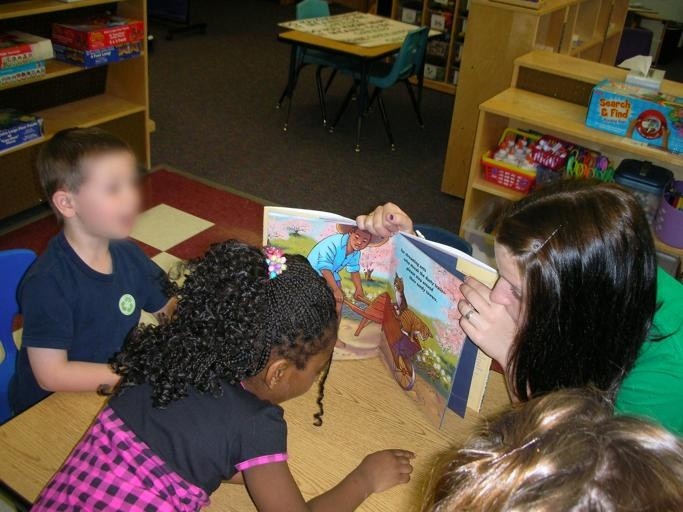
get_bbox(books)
[264,206,498,419]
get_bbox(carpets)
[0,162,279,283]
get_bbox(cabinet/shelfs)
[391,0,471,94]
[0,1,155,236]
[441,0,630,198]
[458,50,681,282]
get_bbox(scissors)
[566,148,615,184]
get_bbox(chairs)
[328,27,432,152]
[275,0,350,139]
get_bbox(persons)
[30,238,416,512]
[624,118,671,150]
[306,223,372,348]
[423,386,683,511]
[9,127,181,416]
[356,176,683,442]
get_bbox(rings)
[465,312,472,320]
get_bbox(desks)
[0,332,514,511]
[276,12,424,140]
